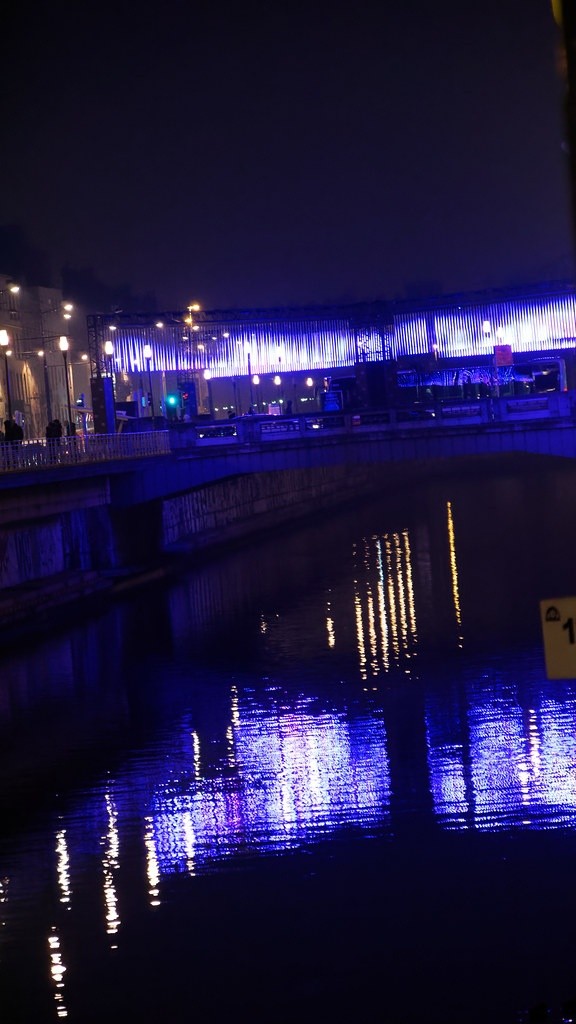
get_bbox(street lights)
[252,374,261,413]
[203,369,215,422]
[274,376,282,414]
[144,345,156,432]
[59,336,74,436]
[105,341,117,434]
[0,329,14,443]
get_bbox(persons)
[424,389,434,402]
[286,400,296,431]
[227,410,236,419]
[45,419,62,463]
[0,419,24,469]
[248,407,254,415]
[64,420,76,457]
